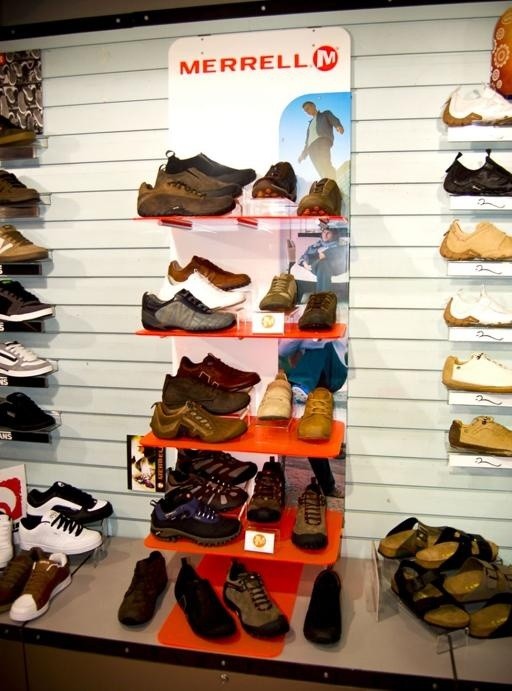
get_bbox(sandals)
[390,560,470,630]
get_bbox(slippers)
[378,517,447,559]
[465,593,512,640]
[444,558,512,602]
[416,526,499,570]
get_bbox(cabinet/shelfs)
[0,117,55,447]
[441,122,511,471]
[130,212,346,568]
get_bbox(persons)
[308,458,344,499]
[298,100,345,180]
[278,339,347,404]
[297,227,348,292]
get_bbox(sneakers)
[442,83,512,127]
[165,150,257,187]
[1,392,56,433]
[150,492,242,548]
[297,388,334,441]
[1,224,48,265]
[442,286,511,328]
[448,416,511,457]
[297,178,342,216]
[175,448,257,485]
[442,353,512,393]
[158,270,246,311]
[247,456,285,523]
[27,482,114,523]
[0,340,54,378]
[1,169,42,207]
[150,400,248,443]
[138,180,235,216]
[0,511,14,571]
[252,162,297,202]
[178,353,260,391]
[440,219,512,262]
[1,280,53,323]
[303,568,342,647]
[10,553,72,622]
[1,548,47,614]
[142,289,236,333]
[168,256,251,291]
[155,164,242,199]
[222,558,290,639]
[258,368,293,422]
[259,274,298,312]
[162,373,250,416]
[1,114,37,149]
[174,557,236,641]
[298,291,336,331]
[19,511,103,556]
[444,148,511,197]
[291,477,327,550]
[166,467,248,511]
[118,551,168,628]
